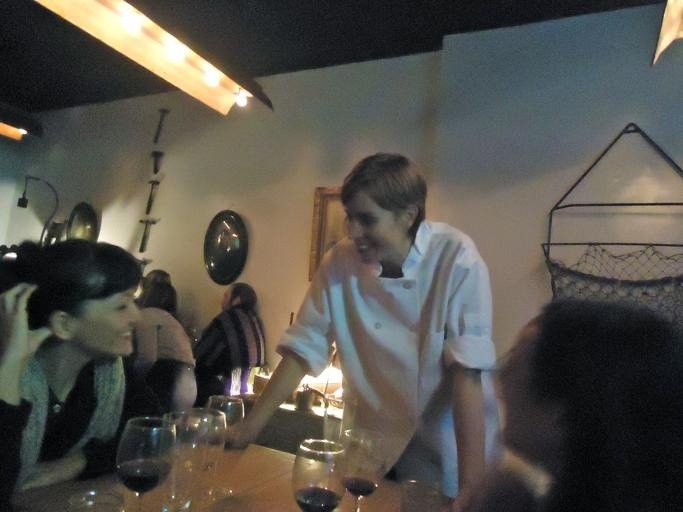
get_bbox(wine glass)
[340,430,385,512]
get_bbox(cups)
[66,396,245,511]
[293,438,348,512]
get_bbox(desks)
[10,393,453,512]
[0,392,453,512]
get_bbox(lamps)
[652,1,682,65]
[0,108,43,143]
[34,0,275,117]
[16,175,66,247]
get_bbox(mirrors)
[204,210,248,285]
[66,202,97,242]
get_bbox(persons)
[190,281,267,409]
[446,290,682,511]
[135,269,173,297]
[212,148,510,511]
[0,239,142,505]
[117,278,200,418]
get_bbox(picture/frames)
[309,185,348,282]
